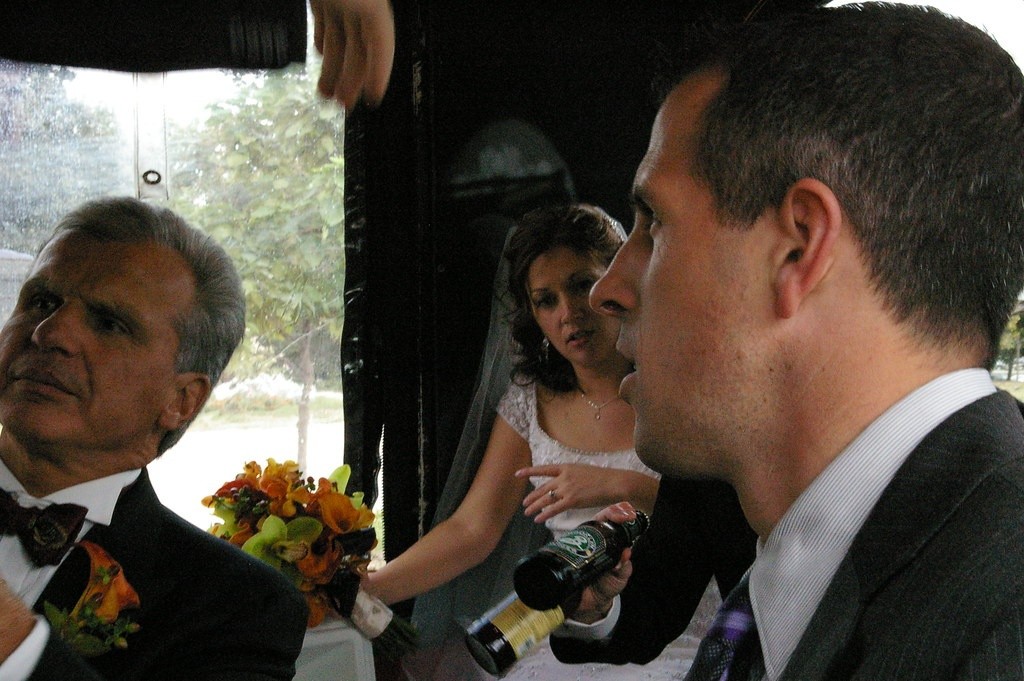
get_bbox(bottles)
[514,510,651,610]
[466,592,586,676]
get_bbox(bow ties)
[0,487,88,569]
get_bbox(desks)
[295,618,375,681]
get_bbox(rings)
[549,489,559,501]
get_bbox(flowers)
[207,455,420,668]
[39,540,142,661]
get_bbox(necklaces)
[574,385,625,421]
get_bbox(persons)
[1,194,311,681]
[343,200,728,681]
[306,0,396,111]
[547,1,1024,681]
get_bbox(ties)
[683,577,757,681]
